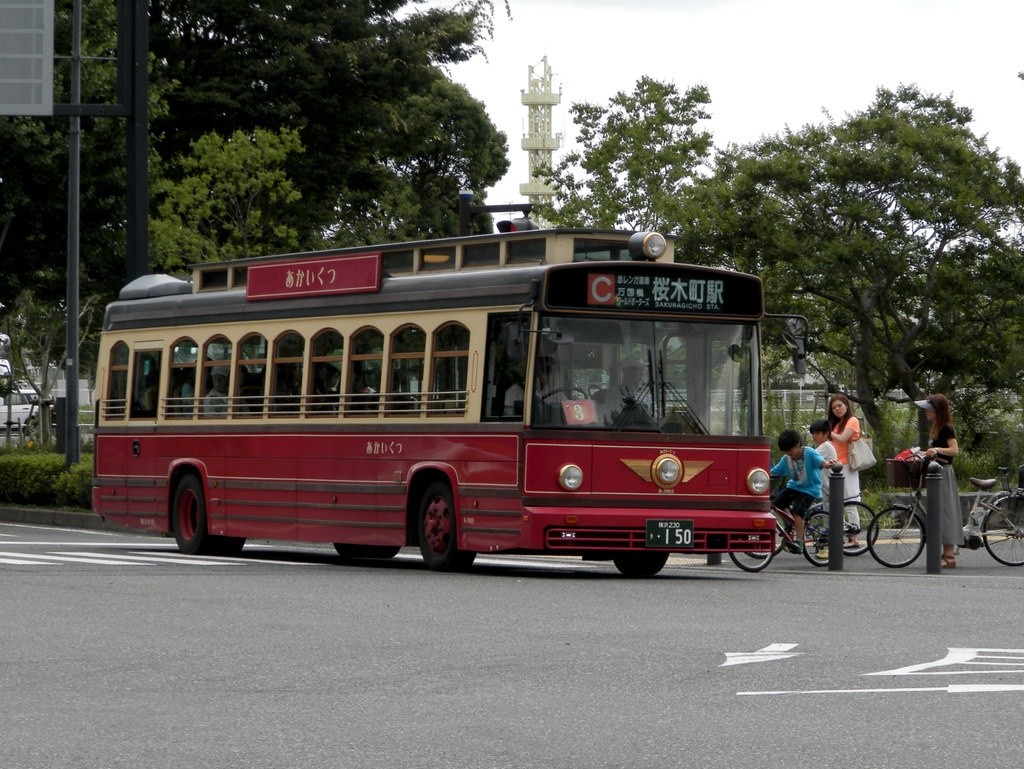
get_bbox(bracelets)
[935,447,939,454]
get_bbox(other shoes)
[843,542,860,548]
[779,522,794,537]
[786,539,803,554]
[814,548,829,559]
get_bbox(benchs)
[245,388,292,416]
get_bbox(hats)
[914,400,935,411]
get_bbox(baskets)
[886,455,926,489]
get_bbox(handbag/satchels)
[844,423,877,472]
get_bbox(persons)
[770,430,839,555]
[504,356,569,415]
[809,420,838,558]
[827,395,861,548]
[611,356,655,421]
[920,393,964,569]
[111,352,418,417]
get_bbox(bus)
[89,223,811,580]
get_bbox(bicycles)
[866,452,1024,570]
[741,500,879,559]
[727,473,832,573]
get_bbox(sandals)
[941,554,956,569]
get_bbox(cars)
[0,389,56,436]
